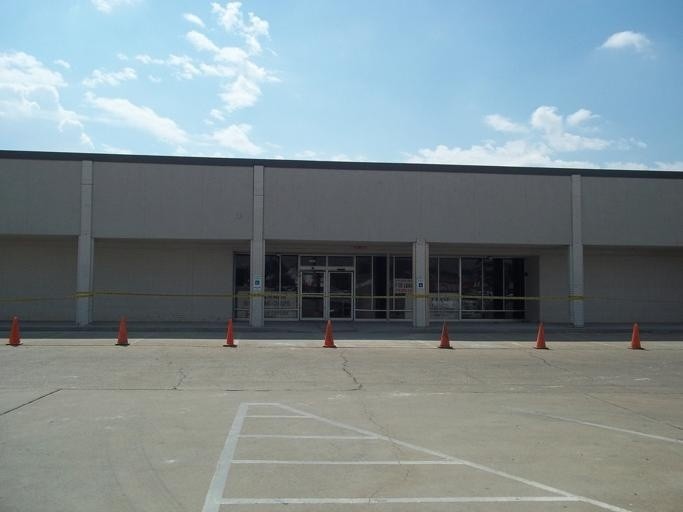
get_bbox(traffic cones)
[115,316,129,346]
[8,315,21,345]
[223,319,237,347]
[534,323,548,350]
[323,319,338,348]
[437,321,451,349]
[628,323,643,350]
